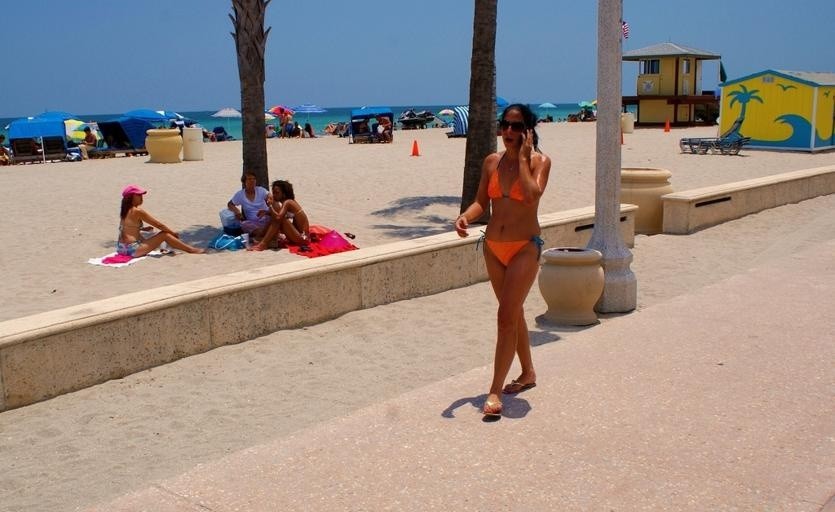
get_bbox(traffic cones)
[661,118,673,133]
[409,139,422,158]
[621,127,625,145]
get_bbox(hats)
[122,187,146,196]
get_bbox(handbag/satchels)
[208,231,248,250]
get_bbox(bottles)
[345,233,355,239]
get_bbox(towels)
[90,242,165,268]
[284,223,358,259]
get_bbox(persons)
[1,127,97,163]
[265,108,318,139]
[219,172,271,243]
[244,181,311,251]
[454,102,551,419]
[116,184,208,258]
[172,120,225,142]
[323,115,394,144]
[537,108,597,123]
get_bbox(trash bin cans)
[621,113,634,134]
[182,127,204,161]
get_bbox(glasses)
[499,120,524,131]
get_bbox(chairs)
[678,116,754,158]
[351,124,371,143]
[214,125,230,141]
[42,136,66,162]
[60,139,114,159]
[8,134,41,165]
[369,123,392,142]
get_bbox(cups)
[234,238,242,249]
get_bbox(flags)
[622,21,630,41]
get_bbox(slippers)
[484,400,501,417]
[502,380,536,394]
[249,246,266,251]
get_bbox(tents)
[5,107,170,163]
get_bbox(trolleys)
[679,117,755,158]
